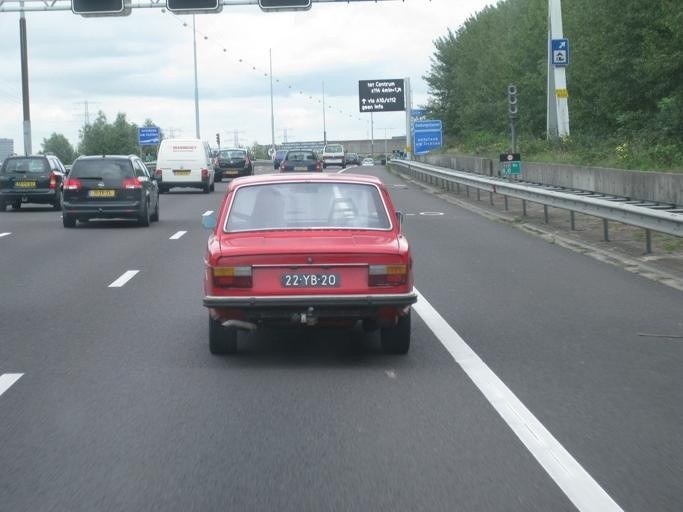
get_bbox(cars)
[198,169,416,354]
[273,143,377,173]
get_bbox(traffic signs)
[500,160,521,175]
[138,126,159,144]
[411,110,429,117]
[358,79,404,111]
[415,131,442,154]
[413,120,441,130]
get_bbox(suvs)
[0,153,66,211]
[61,154,160,228]
[213,147,254,181]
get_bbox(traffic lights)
[71,0,131,15]
[500,154,520,162]
[509,84,520,118]
[259,0,313,11]
[167,0,221,13]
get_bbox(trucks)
[155,139,214,194]
